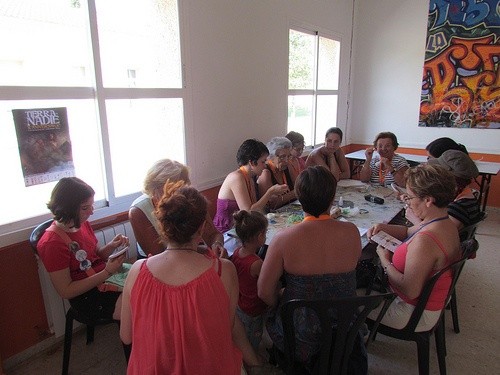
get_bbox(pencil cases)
[364,194,384,205]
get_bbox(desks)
[344,150,500,211]
[223,179,405,289]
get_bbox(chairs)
[365,212,488,375]
[266,292,399,375]
[29,220,132,375]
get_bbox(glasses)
[277,154,293,162]
[403,194,420,203]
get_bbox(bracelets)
[274,184,278,194]
[382,262,394,274]
[391,170,397,176]
[363,163,372,169]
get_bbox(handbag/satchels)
[356,257,387,293]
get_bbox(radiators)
[36,220,138,338]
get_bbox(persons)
[396,136,482,232]
[129,157,270,375]
[258,166,367,359]
[120,181,240,374]
[285,131,342,189]
[213,139,289,234]
[38,177,134,322]
[306,126,352,182]
[226,210,269,355]
[355,164,462,333]
[255,137,298,215]
[360,132,411,192]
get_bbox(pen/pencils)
[226,233,239,239]
[399,201,408,204]
[384,191,394,198]
